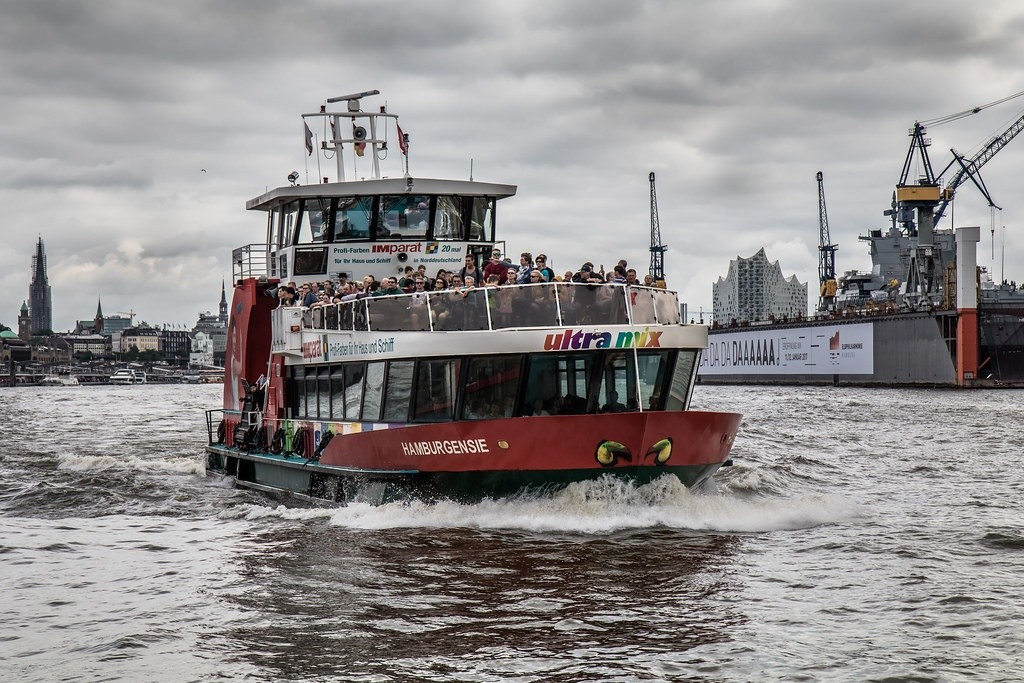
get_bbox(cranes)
[117,308,136,319]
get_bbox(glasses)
[493,252,500,256]
[324,284,329,285]
[323,298,328,299]
[565,274,570,277]
[416,282,424,284]
[303,288,307,289]
[530,275,538,278]
[508,272,515,274]
[387,281,396,283]
[436,281,443,284]
[466,260,471,261]
[298,290,303,292]
[454,280,461,282]
[646,277,652,279]
[536,260,542,263]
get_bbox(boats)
[109,368,148,385]
[202,90,744,509]
[638,118,1024,389]
[37,365,80,386]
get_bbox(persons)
[245,384,265,401]
[461,385,663,419]
[278,248,665,331]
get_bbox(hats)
[337,273,348,277]
[578,265,591,272]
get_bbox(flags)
[331,123,343,149]
[353,124,366,156]
[397,124,409,155]
[305,122,313,156]
